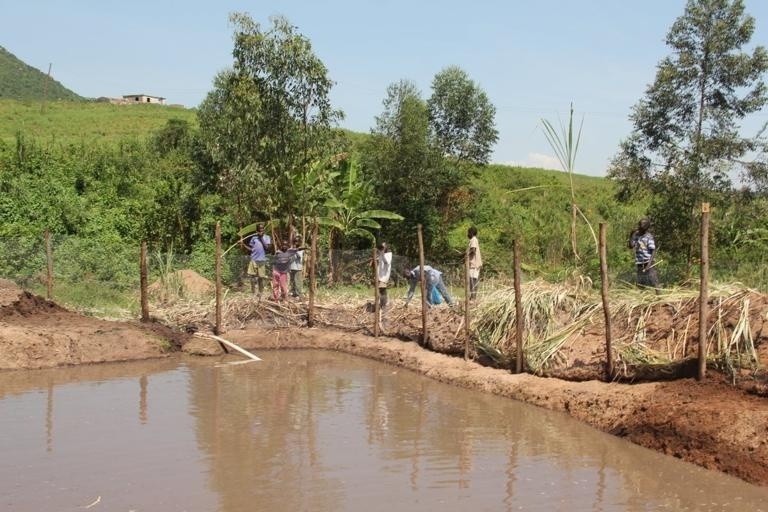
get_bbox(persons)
[401,265,454,308]
[370,241,393,310]
[239,224,270,297]
[269,240,314,304]
[288,236,305,297]
[465,226,484,300]
[628,218,663,300]
